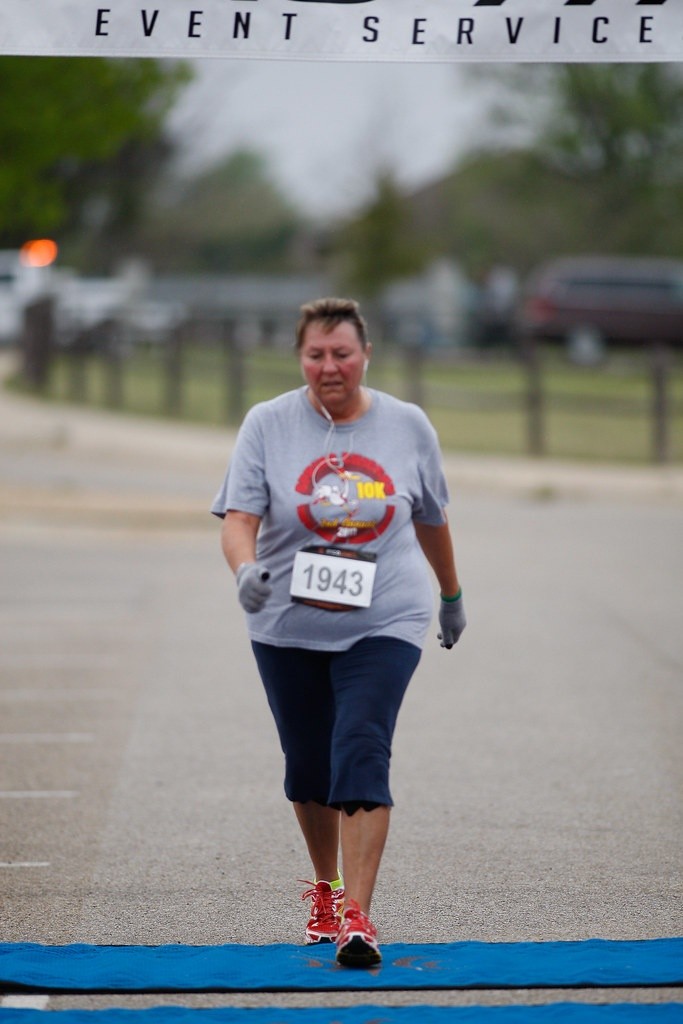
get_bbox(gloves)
[436,590,466,648]
[235,562,272,614]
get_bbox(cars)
[1,247,682,396]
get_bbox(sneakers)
[306,881,344,943]
[333,909,381,966]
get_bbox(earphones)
[363,358,369,372]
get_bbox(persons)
[212,296,467,969]
